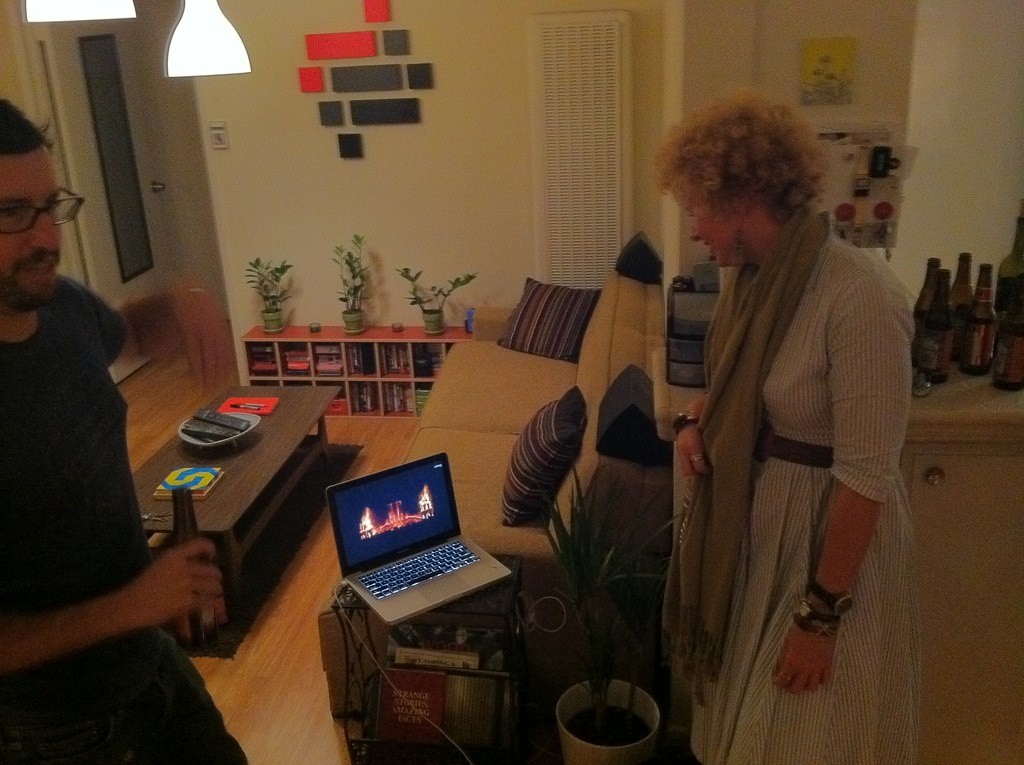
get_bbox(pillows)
[498,279,602,362]
[500,385,587,526]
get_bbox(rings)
[689,454,704,462]
[779,671,791,685]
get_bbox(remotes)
[182,408,252,441]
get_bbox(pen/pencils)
[231,403,266,410]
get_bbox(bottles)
[912,216,1024,391]
[169,488,226,650]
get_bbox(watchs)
[808,580,853,615]
[799,599,840,624]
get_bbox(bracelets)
[672,413,699,434]
[793,613,837,638]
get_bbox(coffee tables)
[133,384,344,623]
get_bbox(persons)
[648,97,923,765]
[0,100,249,765]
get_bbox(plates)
[178,412,262,447]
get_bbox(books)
[380,342,410,375]
[351,381,380,412]
[382,382,414,412]
[348,342,364,373]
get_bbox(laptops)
[324,452,513,624]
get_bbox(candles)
[392,322,403,334]
[309,322,321,335]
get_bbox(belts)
[753,423,834,469]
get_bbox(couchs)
[392,266,650,716]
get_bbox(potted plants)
[536,462,686,765]
[332,233,372,334]
[245,258,296,333]
[396,267,478,335]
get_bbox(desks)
[330,553,527,765]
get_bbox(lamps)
[23,0,251,78]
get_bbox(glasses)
[0,187,86,236]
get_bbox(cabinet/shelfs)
[239,327,473,418]
[654,343,1024,765]
[753,0,923,257]
